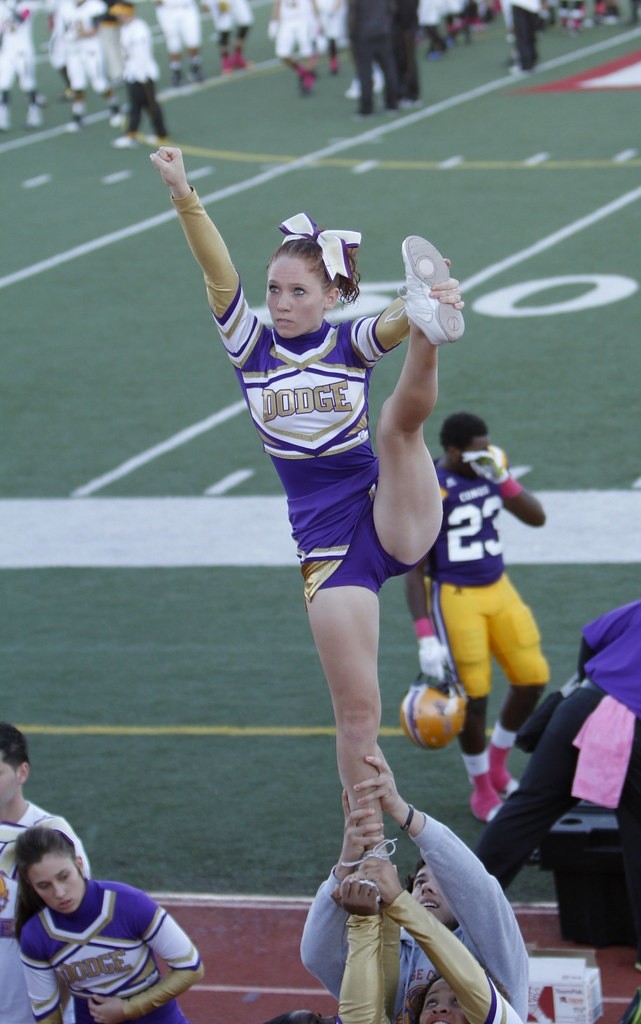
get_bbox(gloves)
[417,635,452,681]
[461,448,510,484]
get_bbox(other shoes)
[343,79,362,100]
[373,71,384,93]
[109,135,136,149]
[298,71,316,94]
[469,789,507,822]
[490,773,521,797]
[146,133,168,146]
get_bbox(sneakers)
[341,837,398,902]
[386,236,465,345]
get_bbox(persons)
[300,740,529,1024]
[0,0,350,150]
[403,409,550,822]
[0,722,205,1024]
[151,146,464,905]
[472,599,641,969]
[338,0,641,116]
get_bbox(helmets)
[399,675,468,749]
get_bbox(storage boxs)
[527,946,604,1024]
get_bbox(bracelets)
[414,617,434,638]
[400,804,414,831]
[495,478,522,500]
